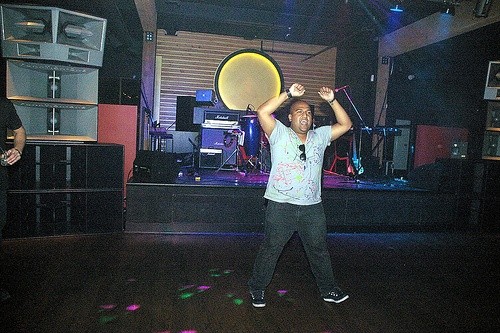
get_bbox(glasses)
[299,144,306,162]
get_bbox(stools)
[151,134,174,154]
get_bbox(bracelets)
[14,148,22,156]
[328,98,336,104]
[286,89,292,99]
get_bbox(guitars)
[351,133,364,175]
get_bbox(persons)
[0,98,26,303]
[249,84,353,307]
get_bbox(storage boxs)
[199,149,222,169]
[486,101,500,132]
[482,132,500,161]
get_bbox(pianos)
[342,125,403,185]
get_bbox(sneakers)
[249,287,266,307]
[322,286,350,303]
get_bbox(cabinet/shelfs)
[4,142,125,239]
[6,59,99,142]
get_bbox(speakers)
[133,150,180,183]
[199,152,222,169]
[175,96,201,132]
[406,164,440,189]
[202,129,236,165]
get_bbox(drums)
[241,114,260,156]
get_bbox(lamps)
[473,0,493,18]
[145,30,154,41]
[390,0,404,12]
[440,0,461,16]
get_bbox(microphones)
[334,84,349,93]
[133,75,141,81]
